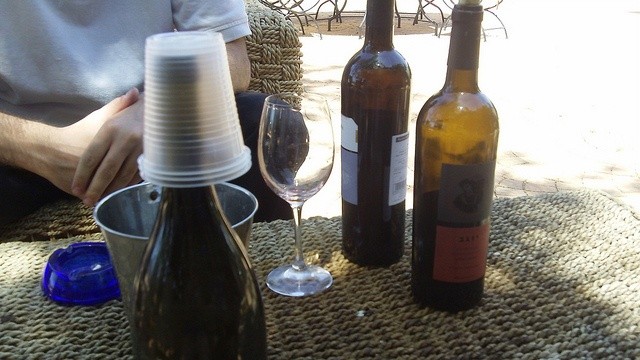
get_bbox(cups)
[138,30,252,189]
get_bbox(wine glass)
[258,92,335,298]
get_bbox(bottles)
[132,53,269,360]
[341,0,412,269]
[411,0,500,312]
